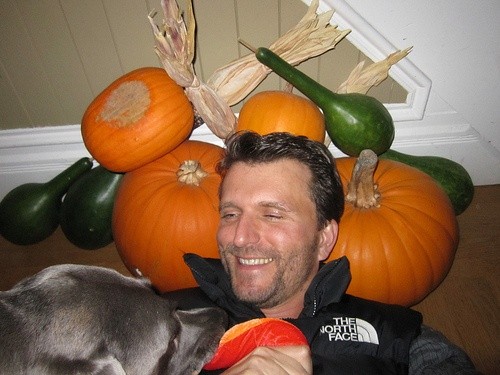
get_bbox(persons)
[161,130,480,375]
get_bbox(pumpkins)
[236,90,326,146]
[80,67,195,174]
[323,149,459,311]
[111,140,231,295]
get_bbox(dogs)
[1,262,230,375]
